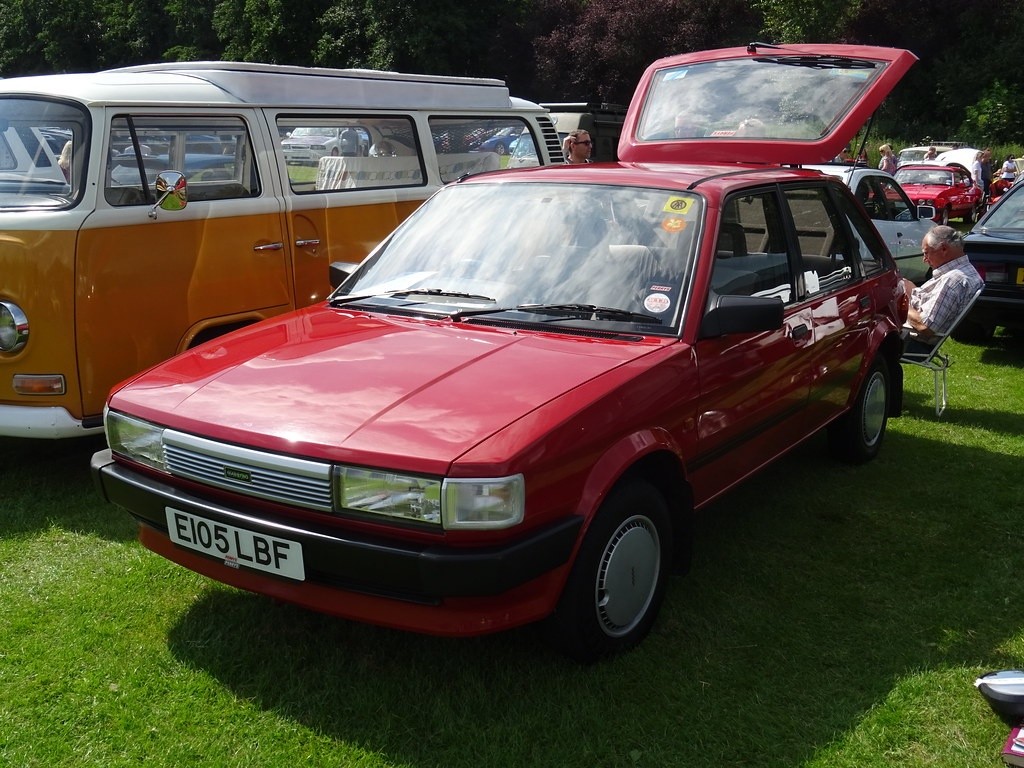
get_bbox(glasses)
[573,140,594,146]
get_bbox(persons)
[563,129,592,164]
[923,147,936,161]
[857,149,868,167]
[1003,154,1017,182]
[58,141,72,184]
[900,225,984,362]
[878,144,897,174]
[981,148,991,195]
[971,151,983,190]
[831,143,851,163]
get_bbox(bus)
[0,58,568,443]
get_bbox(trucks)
[503,101,628,171]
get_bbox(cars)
[84,34,914,661]
[1,119,521,175]
[891,140,962,169]
[935,148,997,176]
[959,177,1024,329]
[886,162,983,225]
[796,167,960,285]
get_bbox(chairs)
[99,150,795,323]
[895,283,986,418]
